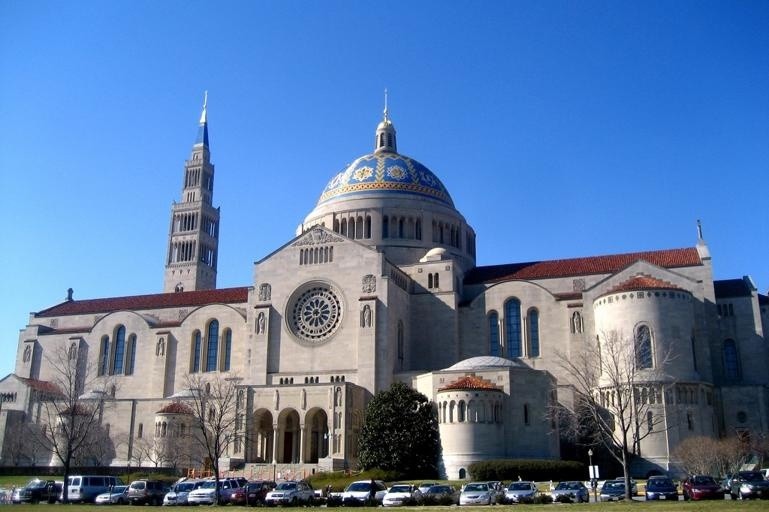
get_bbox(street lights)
[586,449,595,490]
[271,459,276,481]
[125,460,130,484]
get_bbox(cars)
[598,467,769,503]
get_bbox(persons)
[8,484,16,505]
[326,484,333,497]
[368,478,377,507]
[518,475,522,481]
